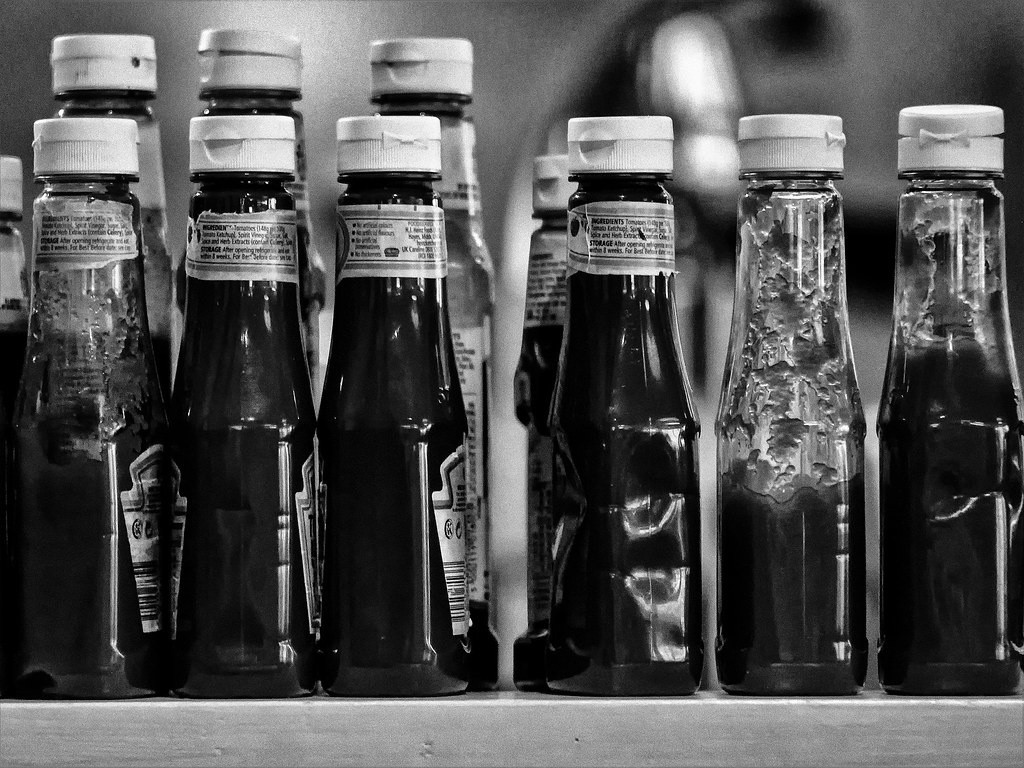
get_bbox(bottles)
[511,154,579,692]
[543,115,709,698]
[161,115,317,700]
[368,36,503,692]
[0,157,32,427]
[877,104,1024,699]
[45,34,178,430]
[714,113,870,699]
[175,29,328,414]
[317,118,471,700]
[3,118,190,700]
